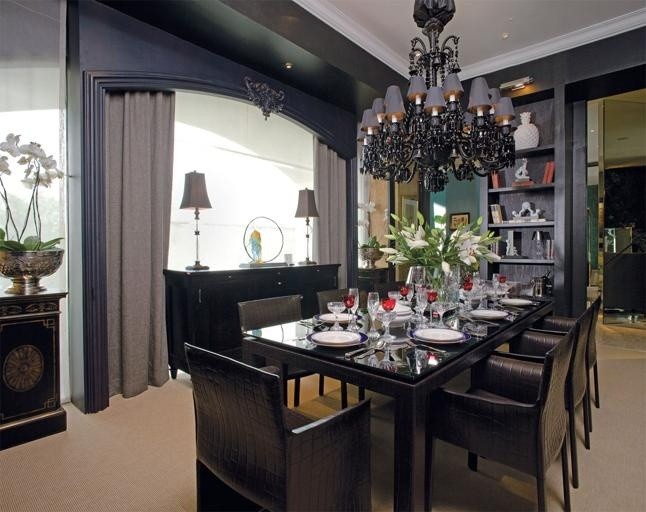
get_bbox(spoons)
[357,341,385,360]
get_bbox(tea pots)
[526,230,546,259]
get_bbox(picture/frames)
[449,212,469,231]
[401,196,419,234]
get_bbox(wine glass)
[492,273,500,300]
[343,295,354,314]
[346,288,361,331]
[415,287,427,327]
[499,282,513,299]
[462,282,474,311]
[434,300,449,328]
[377,341,394,374]
[372,300,400,341]
[367,292,380,339]
[399,286,408,301]
[405,283,414,302]
[425,290,437,327]
[326,302,346,331]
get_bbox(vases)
[359,248,383,269]
[428,269,460,303]
[0,247,62,295]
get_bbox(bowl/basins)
[374,302,413,327]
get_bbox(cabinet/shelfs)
[479,88,572,319]
[161,263,341,379]
[0,290,68,451]
[602,250,646,323]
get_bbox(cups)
[381,299,395,313]
[283,253,293,264]
[366,354,378,370]
[415,348,427,374]
[387,291,402,301]
[427,351,439,366]
[545,239,554,260]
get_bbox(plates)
[312,313,364,324]
[406,328,472,345]
[494,299,540,306]
[463,309,512,319]
[305,331,369,348]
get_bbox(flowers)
[359,201,386,247]
[0,132,64,250]
[379,211,500,296]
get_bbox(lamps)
[498,75,535,92]
[353,0,518,194]
[293,187,320,264]
[178,170,212,271]
